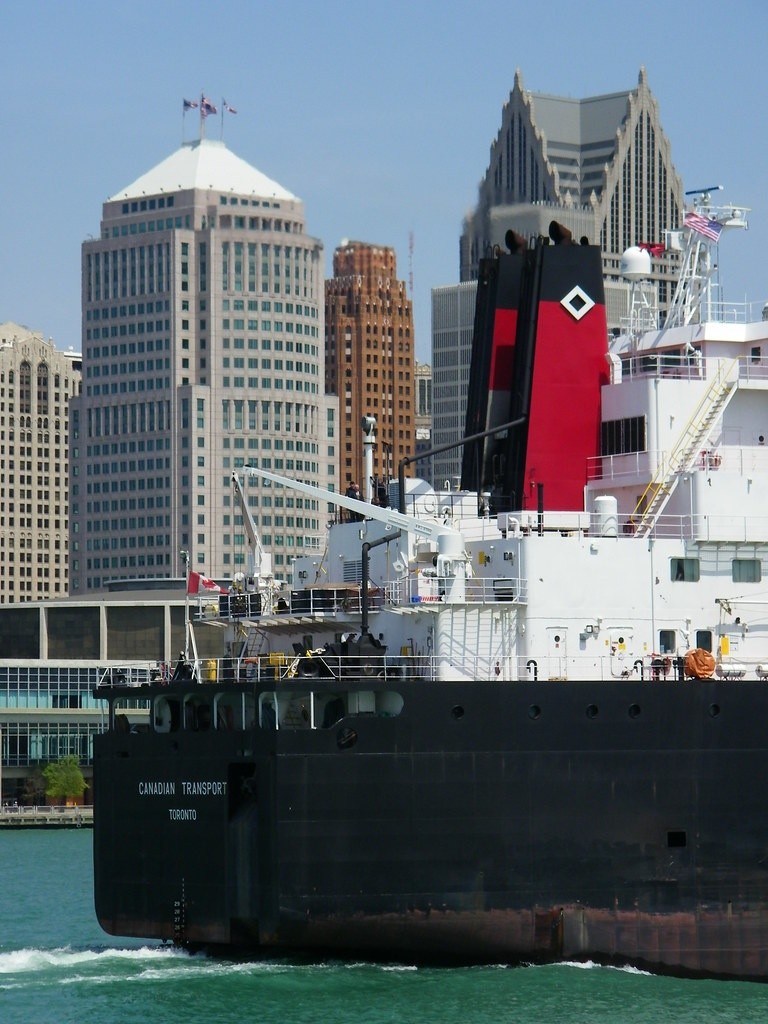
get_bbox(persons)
[222,641,235,679]
[344,482,383,523]
[172,650,186,681]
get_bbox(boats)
[92,186,768,980]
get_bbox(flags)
[683,212,724,243]
[186,570,230,596]
